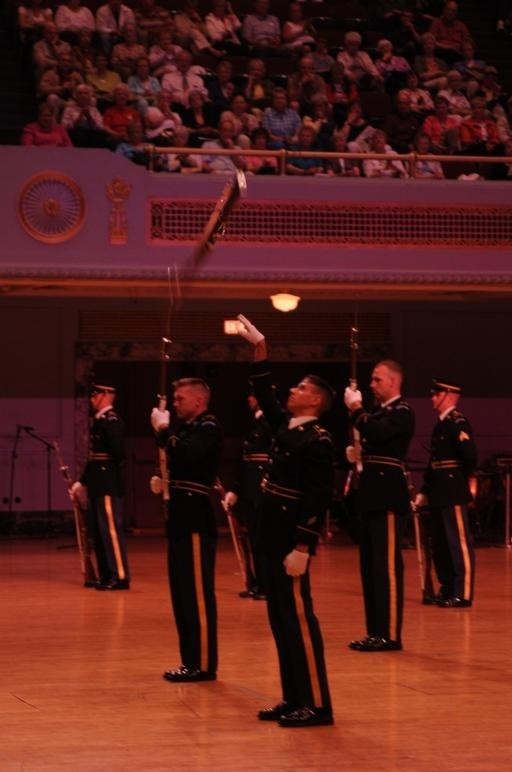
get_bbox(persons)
[220,381,277,601]
[66,383,131,591]
[409,376,484,607]
[149,379,229,685]
[1,2,512,178]
[233,313,343,727]
[343,358,418,650]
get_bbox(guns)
[345,325,363,492]
[405,469,437,603]
[213,482,257,594]
[51,441,102,587]
[157,338,175,521]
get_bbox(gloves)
[150,408,170,432]
[220,491,238,510]
[151,476,162,494]
[346,446,358,463]
[69,482,82,500]
[284,549,309,576]
[344,387,362,407]
[410,493,424,511]
[238,314,264,345]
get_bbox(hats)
[431,378,462,393]
[89,383,115,395]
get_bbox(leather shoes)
[349,636,401,651]
[94,577,129,590]
[240,586,267,599]
[258,701,333,725]
[437,592,470,607]
[164,664,215,681]
